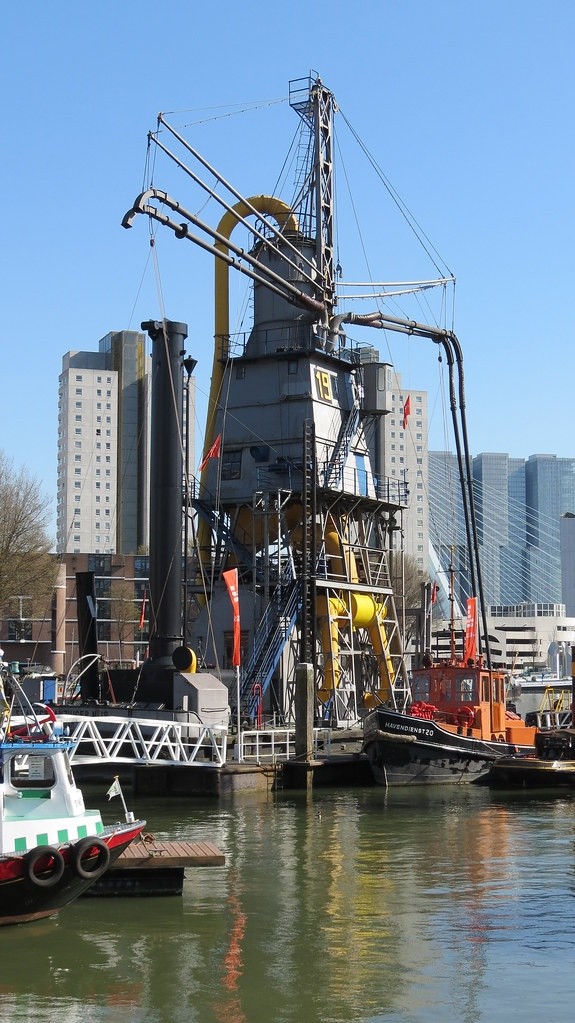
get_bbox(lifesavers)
[453,706,475,727]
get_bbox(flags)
[431,576,437,605]
[105,780,121,801]
[137,590,146,631]
[197,431,222,473]
[402,393,411,431]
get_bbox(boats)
[0,538,574,925]
